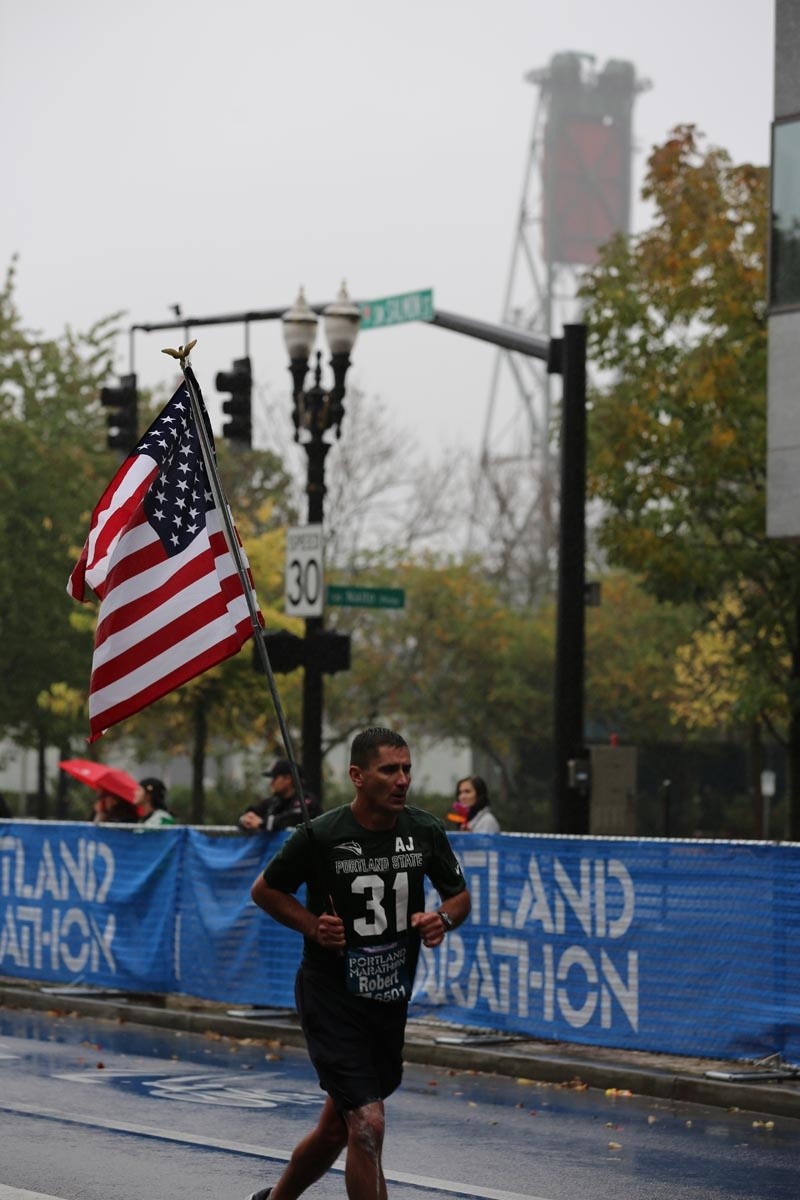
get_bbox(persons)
[89,777,175,828]
[238,758,328,832]
[251,727,470,1200]
[454,775,500,836]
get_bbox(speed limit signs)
[283,524,325,617]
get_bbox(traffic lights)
[99,373,138,464]
[215,356,252,447]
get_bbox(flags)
[65,365,265,744]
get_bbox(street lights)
[247,276,363,809]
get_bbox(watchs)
[438,911,453,932]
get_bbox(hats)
[262,759,302,778]
[140,777,168,810]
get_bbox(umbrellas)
[60,760,148,817]
